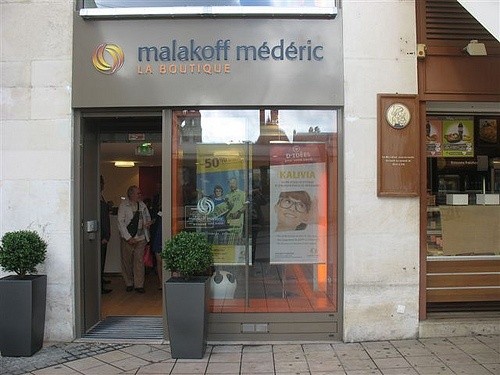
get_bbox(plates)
[480,137,495,144]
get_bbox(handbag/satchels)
[127,210,140,237]
[143,244,154,268]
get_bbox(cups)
[111,206,118,215]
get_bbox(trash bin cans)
[208,269,238,301]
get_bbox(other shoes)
[126,286,133,291]
[103,286,112,294]
[135,288,145,293]
[102,279,111,284]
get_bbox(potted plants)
[159,231,213,359]
[0,231,47,359]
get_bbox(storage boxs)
[447,194,468,206]
[476,194,499,204]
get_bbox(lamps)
[114,162,135,168]
[464,40,487,56]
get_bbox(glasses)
[215,189,223,192]
[278,197,309,214]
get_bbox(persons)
[117,185,162,293]
[241,185,267,270]
[100,175,114,294]
[207,177,251,245]
[273,191,312,232]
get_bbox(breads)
[430,135,438,140]
[479,126,497,141]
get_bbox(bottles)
[426,120,431,136]
[458,122,463,139]
